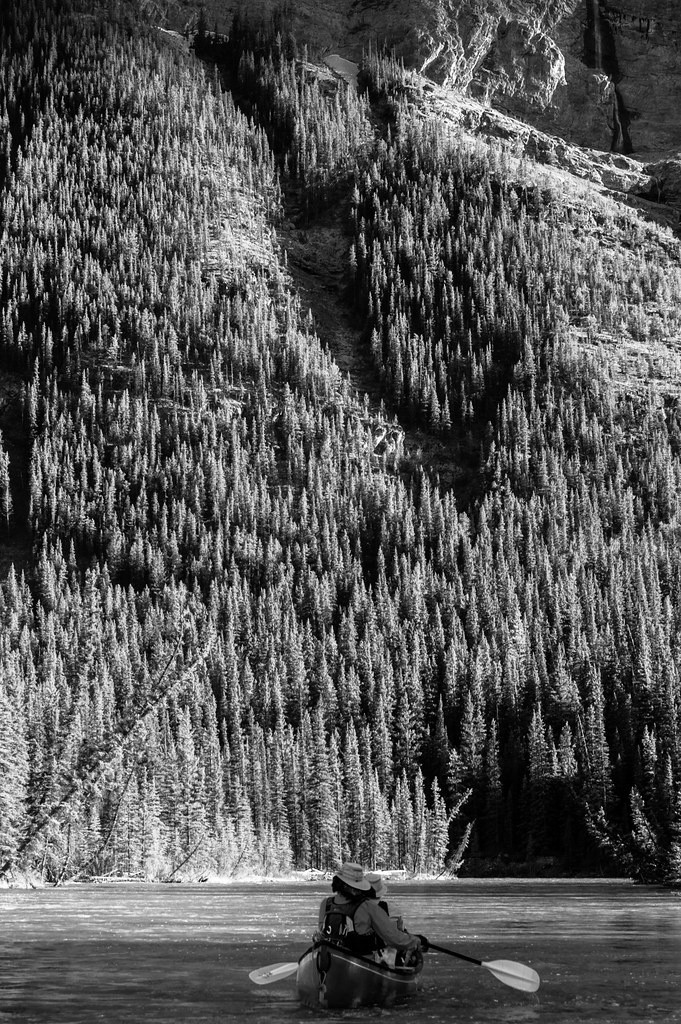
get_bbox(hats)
[335,863,371,890]
[365,873,386,897]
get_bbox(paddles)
[249,948,314,986]
[426,941,540,992]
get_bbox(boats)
[291,931,425,1012]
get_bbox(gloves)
[415,935,429,953]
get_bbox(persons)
[318,864,422,968]
[365,875,390,918]
